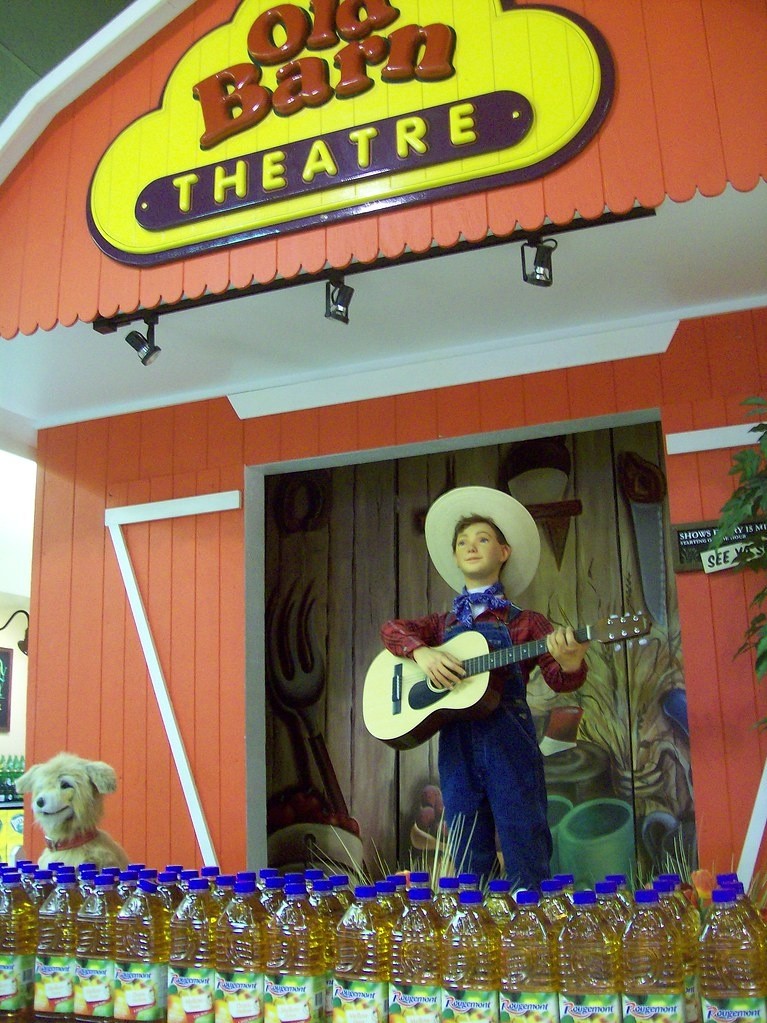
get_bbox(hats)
[424,486,541,598]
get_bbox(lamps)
[125,316,163,366]
[324,277,354,325]
[520,236,558,288]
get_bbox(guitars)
[362,611,652,752]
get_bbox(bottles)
[0,755,25,801]
[0,862,767,1023]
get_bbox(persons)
[381,485,589,890]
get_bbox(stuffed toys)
[13,752,132,867]
